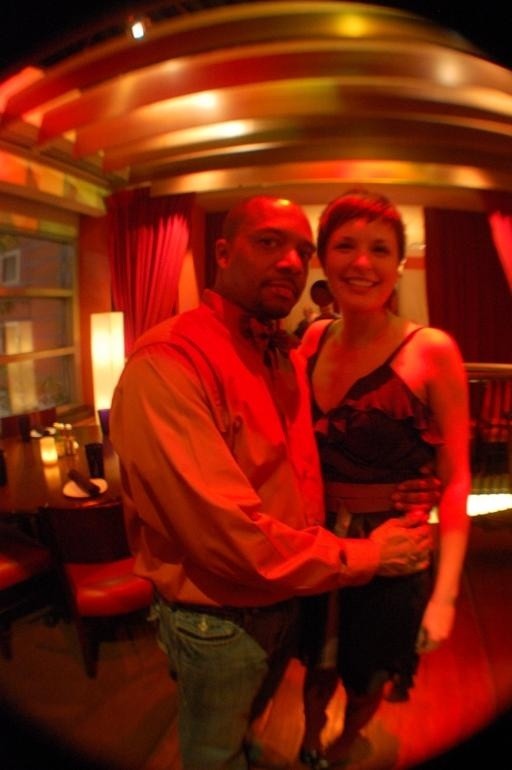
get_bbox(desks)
[0,479,128,624]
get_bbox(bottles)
[53,421,74,457]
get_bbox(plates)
[61,477,110,500]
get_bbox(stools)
[2,534,65,654]
[63,552,160,679]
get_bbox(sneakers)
[243,732,288,769]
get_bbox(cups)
[39,436,58,465]
[83,443,107,477]
[98,408,109,437]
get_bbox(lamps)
[86,306,127,413]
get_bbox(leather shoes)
[298,747,349,769]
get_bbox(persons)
[286,189,474,769]
[107,194,443,769]
